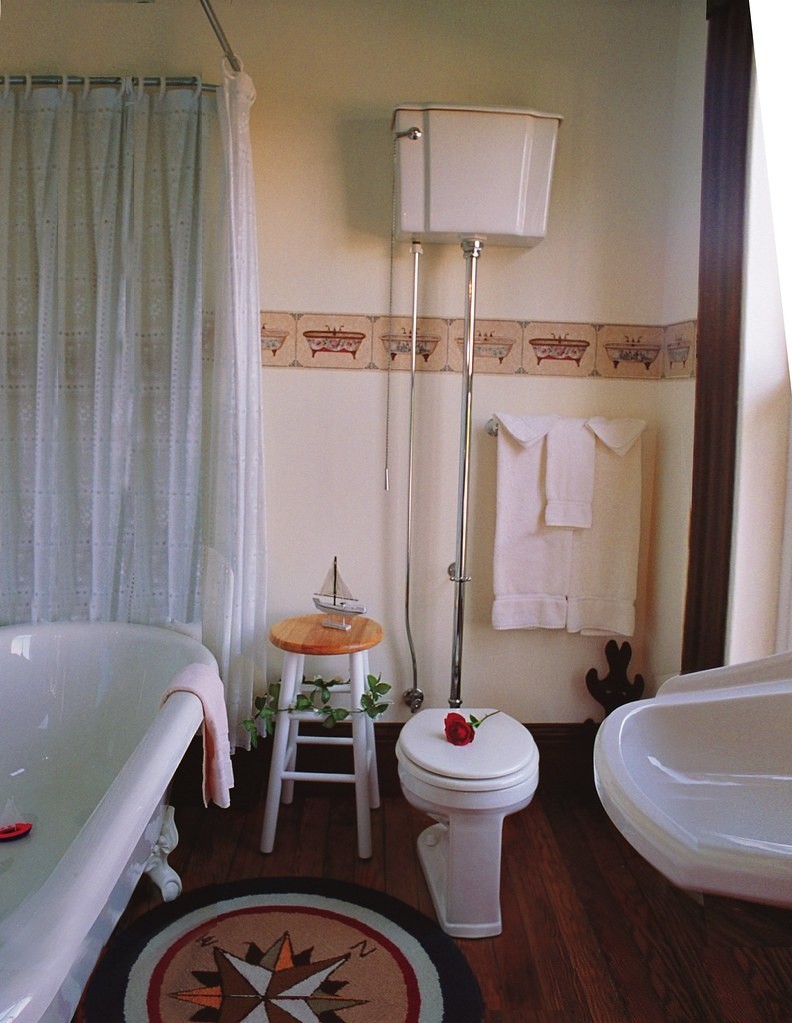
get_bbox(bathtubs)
[1,619,222,1021]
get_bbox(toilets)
[395,705,543,940]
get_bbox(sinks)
[592,648,791,907]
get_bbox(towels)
[490,409,650,639]
[161,661,236,809]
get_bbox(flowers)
[444,710,501,746]
[260,612,383,858]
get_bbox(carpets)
[84,875,485,1023]
